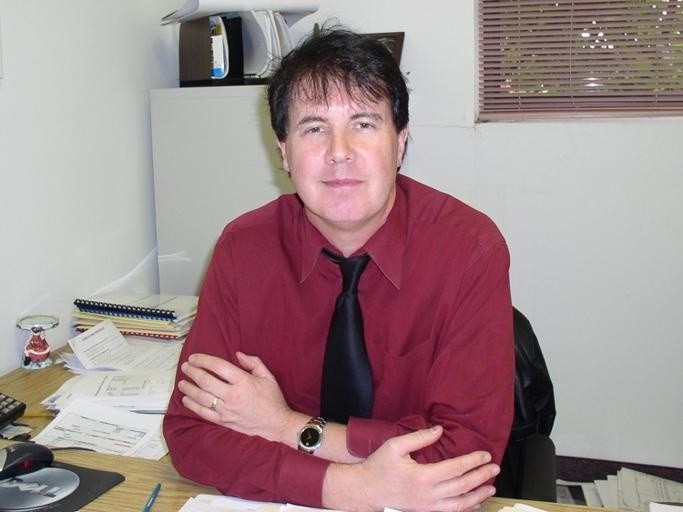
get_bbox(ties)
[317,247,375,422]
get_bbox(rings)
[211,397,218,409]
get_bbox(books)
[176,9,299,89]
[577,466,683,512]
[71,287,202,343]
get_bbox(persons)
[160,16,521,511]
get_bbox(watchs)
[297,415,327,454]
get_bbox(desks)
[1,344,653,512]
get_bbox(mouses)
[0,442,54,479]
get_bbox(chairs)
[495,313,559,500]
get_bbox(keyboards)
[0,391,26,430]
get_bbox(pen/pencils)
[143,483,161,512]
[21,412,56,417]
